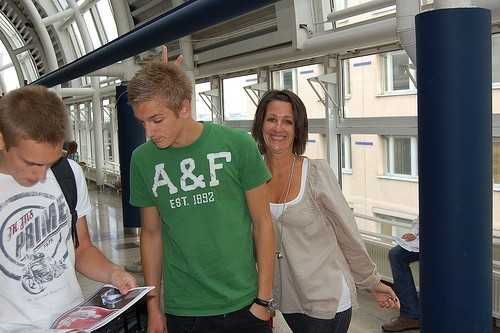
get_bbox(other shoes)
[381,317,420,333]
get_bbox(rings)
[388,300,394,305]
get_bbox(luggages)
[99,303,146,333]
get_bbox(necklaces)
[276,152,296,259]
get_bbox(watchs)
[252,296,276,314]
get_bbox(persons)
[381,215,419,333]
[160,45,401,333]
[129,60,277,333]
[0,86,138,333]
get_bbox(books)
[0,284,155,333]
